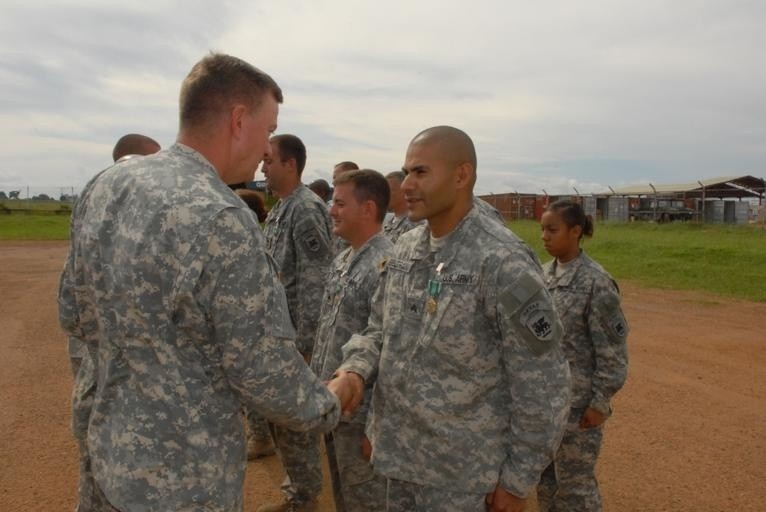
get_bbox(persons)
[539,197,630,511]
[321,123,572,511]
[308,168,395,512]
[227,159,428,459]
[259,134,332,512]
[56,49,358,512]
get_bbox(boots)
[247,419,277,460]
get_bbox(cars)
[629,205,695,224]
